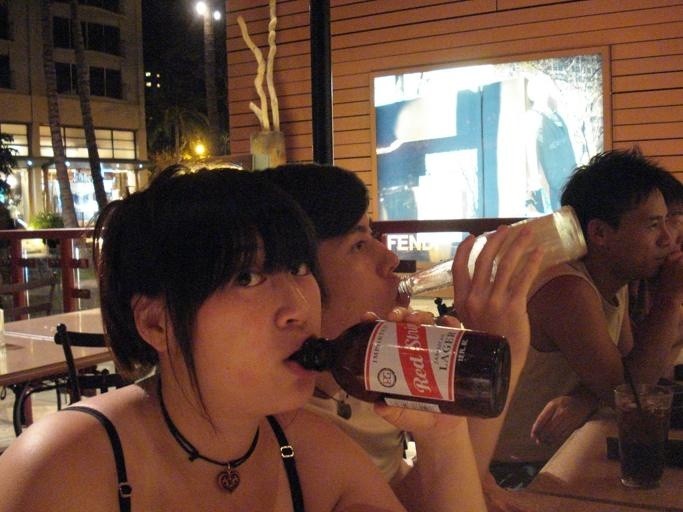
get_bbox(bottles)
[294,318,510,418]
[399,206,588,300]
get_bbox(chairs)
[15,324,132,438]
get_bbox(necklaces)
[313,384,355,422]
[155,372,262,493]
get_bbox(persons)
[628,165,683,363]
[266,159,546,511]
[486,145,682,487]
[0,164,487,512]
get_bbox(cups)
[612,383,673,489]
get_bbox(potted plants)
[31,211,65,255]
